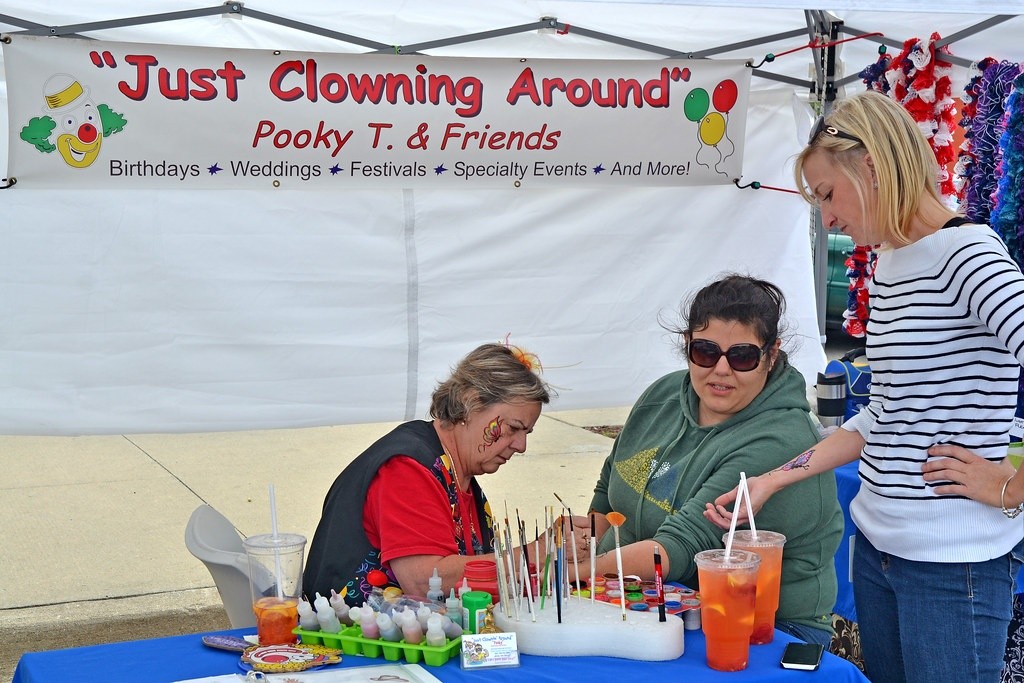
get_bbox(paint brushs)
[492,493,627,623]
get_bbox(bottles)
[455,560,500,605]
[521,563,541,597]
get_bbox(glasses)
[686,335,770,371]
[806,115,863,146]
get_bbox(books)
[781,642,825,671]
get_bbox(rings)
[581,527,591,550]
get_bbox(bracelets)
[1001,475,1024,519]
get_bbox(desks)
[834,459,1024,623]
[11,627,874,683]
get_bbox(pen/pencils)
[653,546,667,622]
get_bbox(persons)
[540,277,844,652]
[703,93,1024,683]
[303,344,592,613]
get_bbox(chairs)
[184,503,286,630]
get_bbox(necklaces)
[444,443,476,537]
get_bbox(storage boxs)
[292,625,474,667]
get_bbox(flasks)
[816,372,847,431]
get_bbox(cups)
[694,548,763,672]
[722,529,786,645]
[242,533,307,645]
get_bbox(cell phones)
[781,642,825,671]
